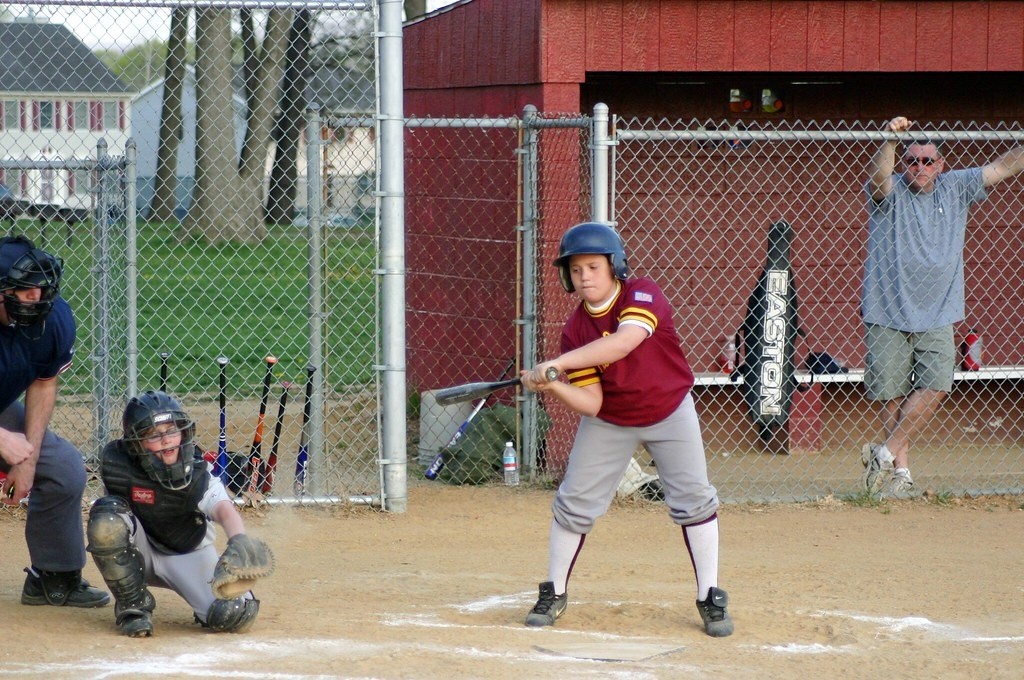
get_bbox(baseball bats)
[435,366,560,408]
[424,356,516,481]
[161,352,317,498]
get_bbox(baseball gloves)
[210,532,276,601]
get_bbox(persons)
[861,114,1024,500]
[85,390,277,640]
[0,235,112,609]
[523,223,735,638]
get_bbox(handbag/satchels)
[222,452,248,498]
[730,220,814,445]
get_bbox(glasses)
[905,156,938,166]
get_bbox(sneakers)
[525,582,568,628]
[121,616,154,639]
[889,472,916,500]
[20,566,110,608]
[695,586,733,638]
[861,442,892,499]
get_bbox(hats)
[805,352,849,374]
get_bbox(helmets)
[122,389,196,492]
[552,222,628,292]
[0,233,64,343]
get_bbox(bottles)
[961,328,982,372]
[503,442,519,486]
[722,335,736,372]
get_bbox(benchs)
[695,364,1024,457]
